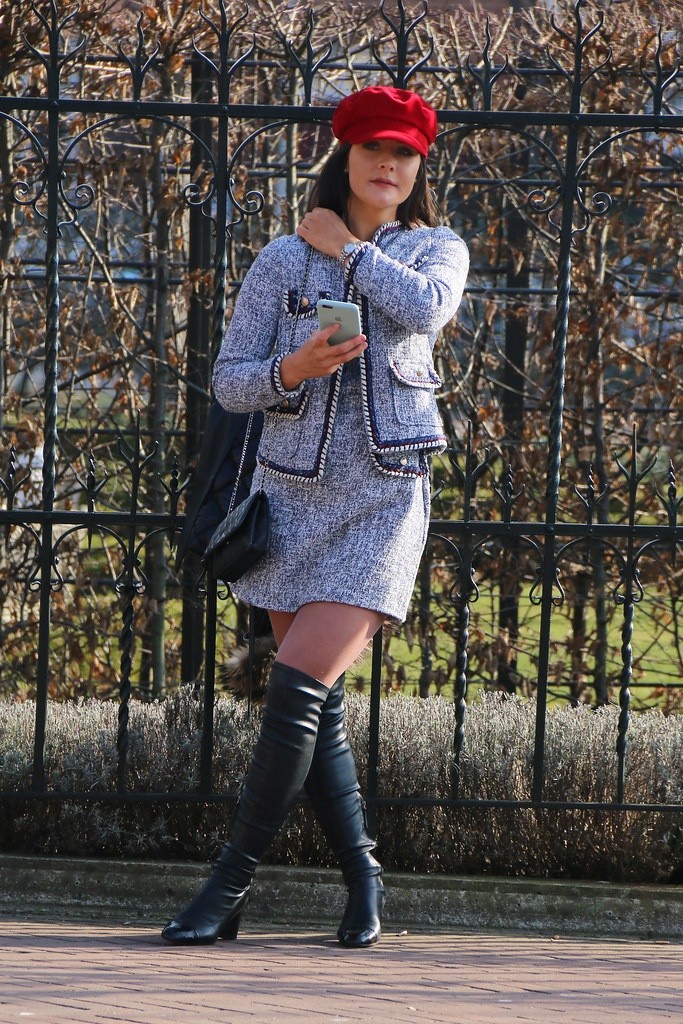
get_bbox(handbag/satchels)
[200,488,272,583]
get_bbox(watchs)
[339,240,364,268]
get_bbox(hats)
[331,85,438,159]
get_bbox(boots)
[305,671,388,947]
[158,660,331,946]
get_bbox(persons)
[157,88,471,942]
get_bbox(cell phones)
[317,300,364,358]
